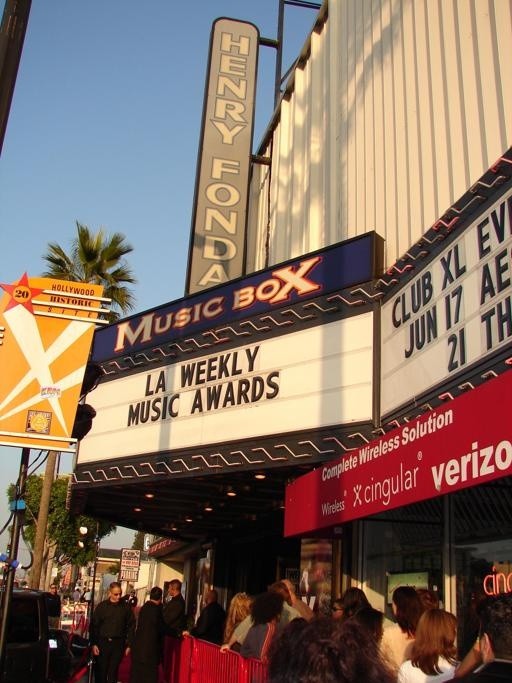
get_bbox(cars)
[48,628,75,682]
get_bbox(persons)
[47,571,512,683]
[300,557,315,594]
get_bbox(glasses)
[331,607,344,611]
[111,592,122,596]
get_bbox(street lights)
[79,525,101,641]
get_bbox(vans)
[0,589,50,681]
